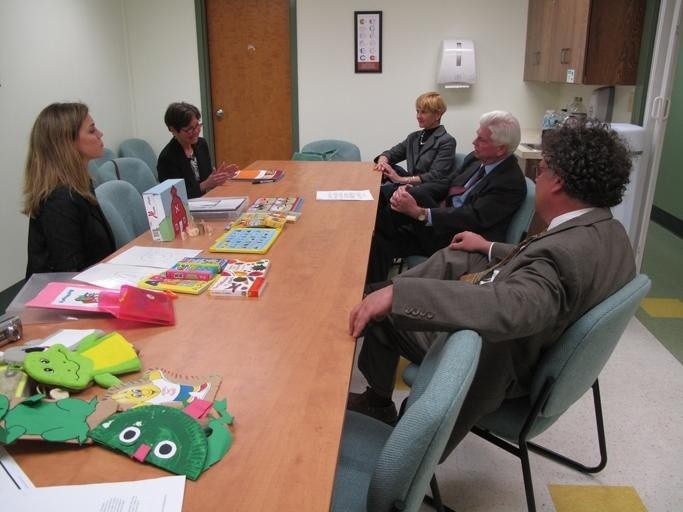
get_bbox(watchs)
[417,208,426,223]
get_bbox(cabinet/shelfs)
[523,0,646,86]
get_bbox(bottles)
[543,110,561,131]
[568,96,589,125]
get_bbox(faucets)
[558,114,581,129]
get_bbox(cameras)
[0,313,24,347]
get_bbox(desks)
[0,161,383,512]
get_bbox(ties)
[436,162,486,209]
[456,228,548,282]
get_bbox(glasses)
[175,123,202,134]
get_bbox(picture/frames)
[354,11,382,73]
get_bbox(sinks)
[528,142,541,150]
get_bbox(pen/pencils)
[252,180,275,184]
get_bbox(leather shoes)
[346,391,397,424]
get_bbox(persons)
[348,121,638,429]
[23,103,116,281]
[157,103,238,197]
[373,93,456,209]
[367,111,527,292]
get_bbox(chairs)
[301,140,361,160]
[118,138,158,183]
[397,176,536,273]
[95,181,150,251]
[93,157,159,198]
[453,153,467,171]
[398,273,651,512]
[328,330,484,511]
[87,146,117,191]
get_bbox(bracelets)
[408,175,414,184]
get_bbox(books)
[229,170,284,180]
[222,259,270,275]
[188,197,304,221]
[208,276,267,295]
[137,269,221,295]
[25,282,115,313]
